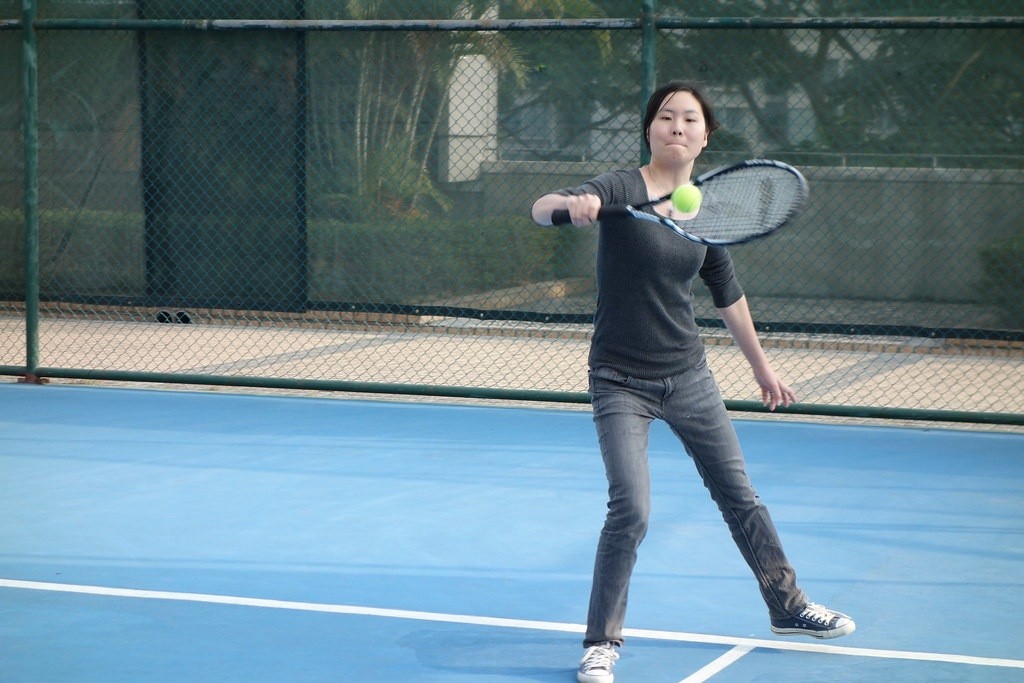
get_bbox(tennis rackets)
[550,158,810,248]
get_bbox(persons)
[530,80,856,683]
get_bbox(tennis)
[671,183,702,213]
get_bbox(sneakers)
[770,601,855,639]
[577,642,620,683]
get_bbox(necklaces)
[647,165,674,218]
